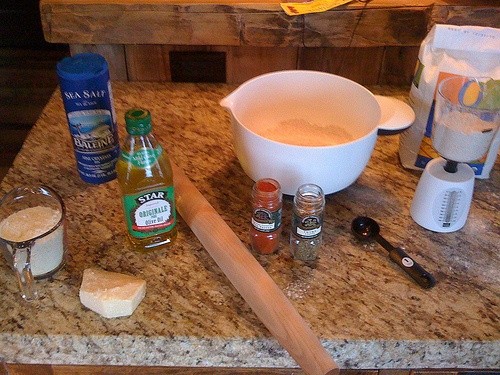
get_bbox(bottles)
[116,107,177,251]
[248,178,326,260]
[55,50,119,185]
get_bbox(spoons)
[351,216,436,290]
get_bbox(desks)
[0,81,500,375]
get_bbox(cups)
[0,182,66,303]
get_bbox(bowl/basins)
[218,70,415,195]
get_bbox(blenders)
[410,75,500,235]
